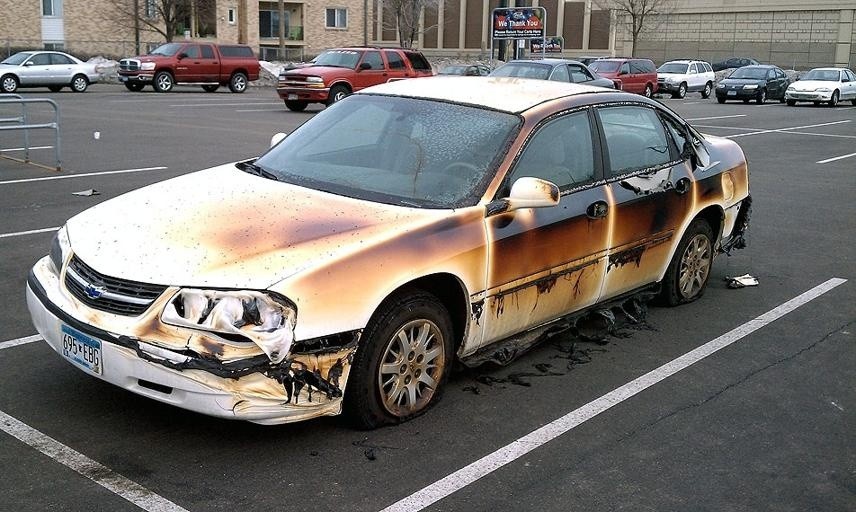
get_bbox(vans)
[585,57,658,98]
[116,42,260,92]
[657,58,716,99]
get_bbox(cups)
[94,130,100,139]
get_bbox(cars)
[277,45,433,111]
[492,59,615,89]
[435,65,490,76]
[26,77,749,432]
[715,64,790,104]
[785,68,855,108]
[711,57,760,72]
[1,51,99,93]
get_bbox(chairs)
[524,135,576,184]
[606,132,648,172]
